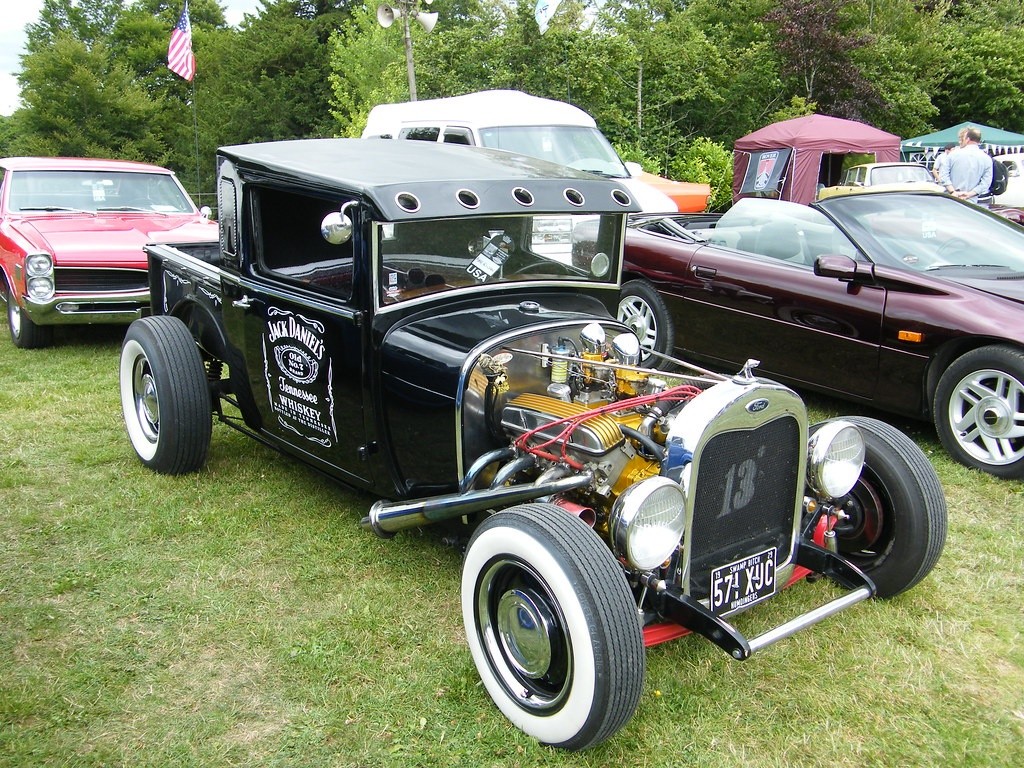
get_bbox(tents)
[900,118,1024,167]
[728,111,900,202]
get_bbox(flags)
[166,1,196,83]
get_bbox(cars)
[119,140,949,754]
[0,157,219,348]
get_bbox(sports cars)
[605,193,1024,478]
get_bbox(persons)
[931,126,994,207]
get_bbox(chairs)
[754,219,815,264]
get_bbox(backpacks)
[989,157,1008,195]
